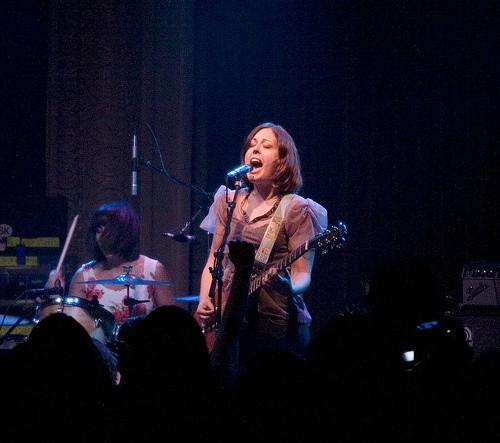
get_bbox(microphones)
[161,232,201,245]
[226,164,252,178]
[131,123,139,196]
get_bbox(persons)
[197,122,319,358]
[69,201,174,332]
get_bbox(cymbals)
[76,275,175,290]
[166,296,206,301]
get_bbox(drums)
[33,295,104,336]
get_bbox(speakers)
[462,276,500,307]
[449,311,500,357]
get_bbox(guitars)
[192,223,347,349]
